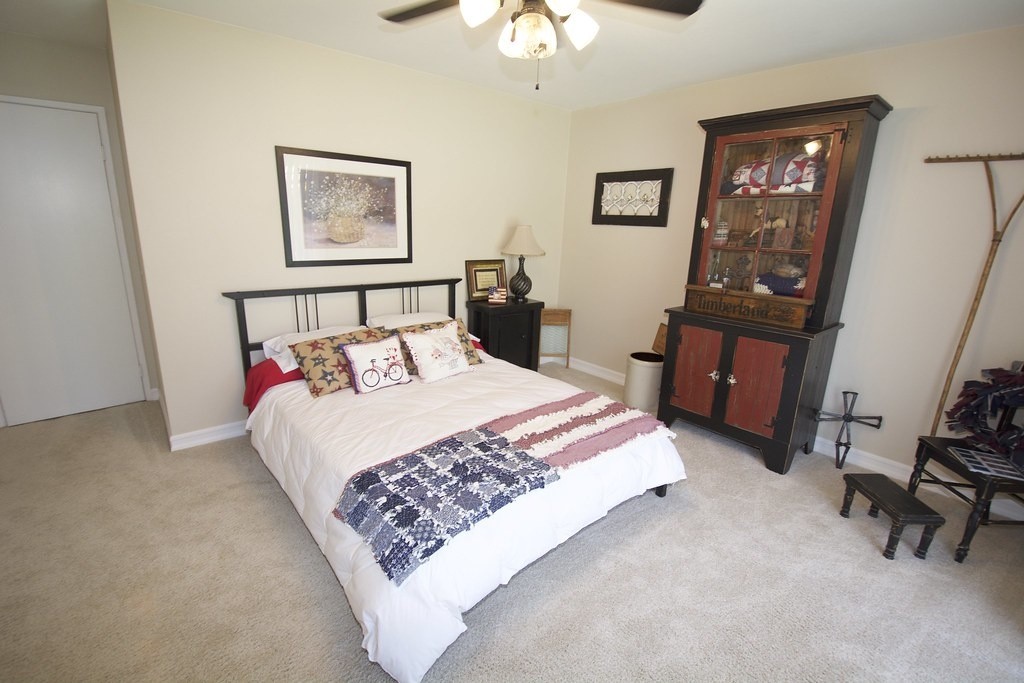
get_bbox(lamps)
[500,225,546,303]
[458,0,600,60]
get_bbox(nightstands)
[466,296,545,372]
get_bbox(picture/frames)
[488,286,507,305]
[464,258,509,302]
[274,145,413,269]
[591,168,675,227]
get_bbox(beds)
[221,278,689,683]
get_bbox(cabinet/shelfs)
[653,91,894,475]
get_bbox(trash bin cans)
[622,352,665,411]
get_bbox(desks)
[907,435,1024,563]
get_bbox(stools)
[838,473,946,561]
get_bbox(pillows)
[402,321,476,384]
[262,324,370,375]
[386,317,485,375]
[338,333,412,395]
[287,326,388,399]
[365,312,481,342]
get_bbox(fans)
[376,0,706,30]
